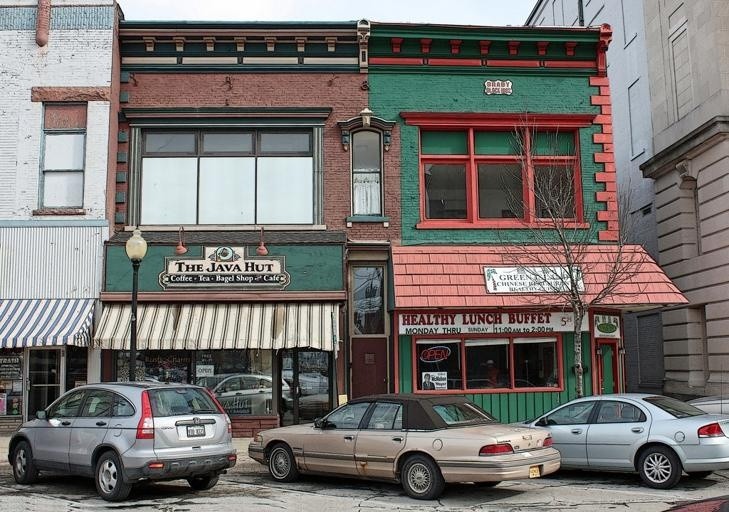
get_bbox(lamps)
[256,226,270,257]
[175,224,188,255]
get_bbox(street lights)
[125,227,147,382]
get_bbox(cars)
[503,392,729,486]
[683,394,729,417]
[247,392,571,498]
[140,371,328,417]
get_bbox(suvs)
[7,377,240,498]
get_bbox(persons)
[423,374,435,390]
